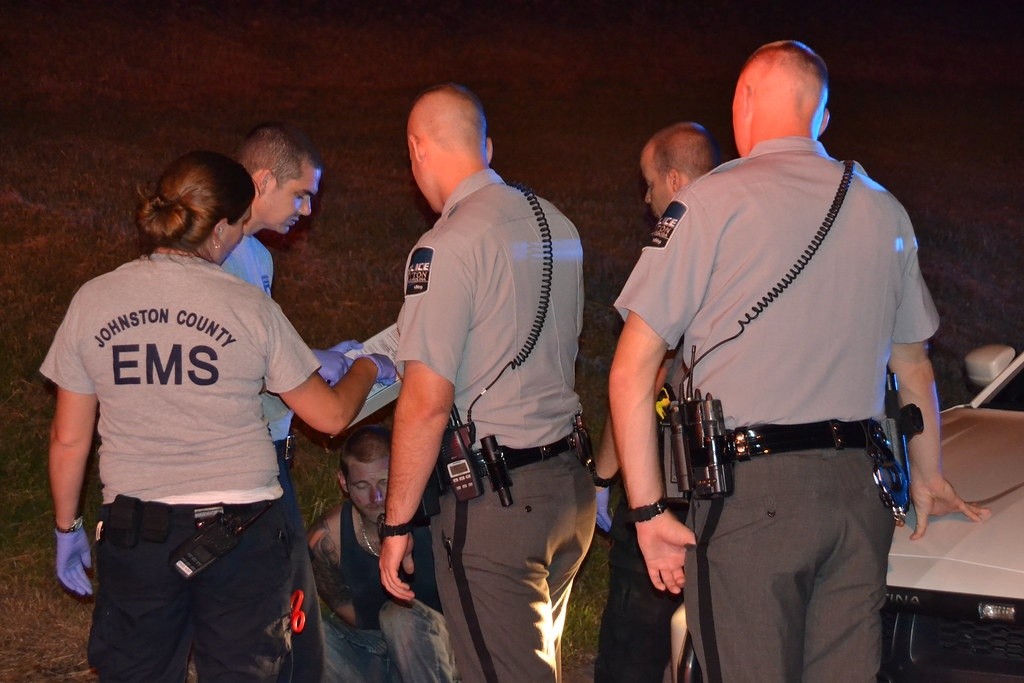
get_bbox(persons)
[36,151,402,683]
[591,122,730,682]
[209,125,366,683]
[301,426,459,683]
[609,41,993,682]
[376,83,600,683]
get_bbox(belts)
[472,430,578,476]
[728,420,887,462]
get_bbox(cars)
[884,344,1023,683]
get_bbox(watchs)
[54,514,84,534]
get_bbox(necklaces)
[358,511,383,558]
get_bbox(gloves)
[53,527,94,596]
[328,339,364,355]
[359,352,396,386]
[310,349,354,386]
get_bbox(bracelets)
[376,514,416,538]
[593,462,622,488]
[628,497,670,524]
